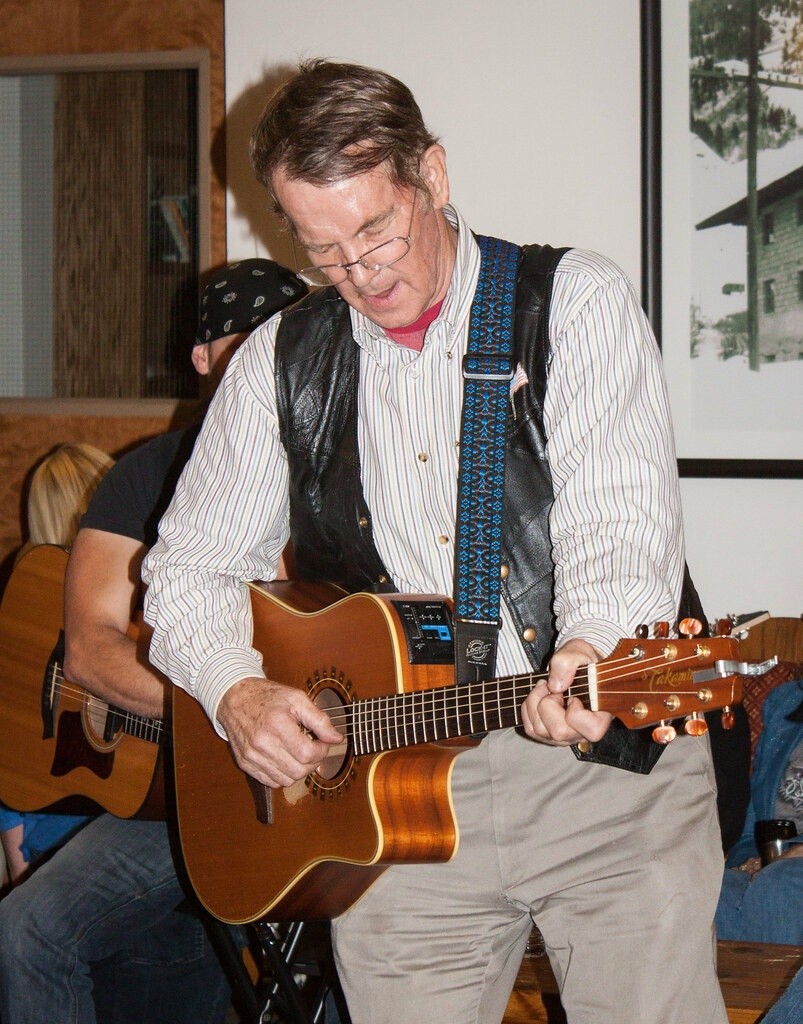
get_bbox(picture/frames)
[640,1,803,481]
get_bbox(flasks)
[756,818,798,869]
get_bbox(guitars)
[0,543,176,823]
[169,573,779,927]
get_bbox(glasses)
[294,157,421,287]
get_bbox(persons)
[1,257,803,1024]
[138,60,724,1024]
